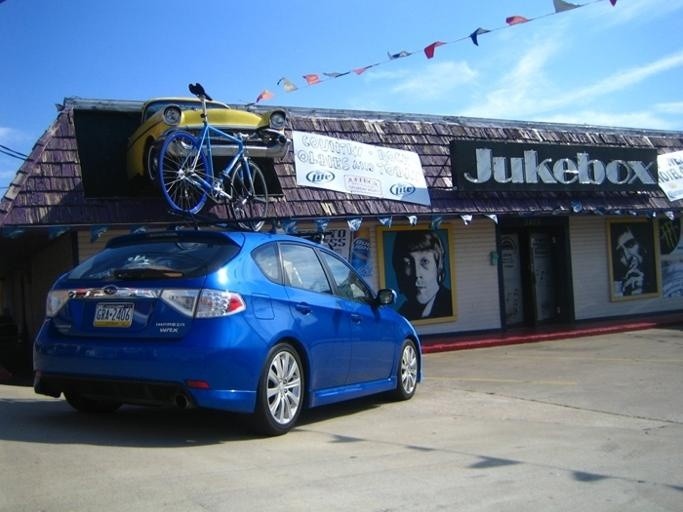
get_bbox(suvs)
[124,97,293,189]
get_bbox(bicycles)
[157,83,270,232]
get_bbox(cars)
[33,230,424,437]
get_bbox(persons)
[391,231,453,320]
[613,226,644,296]
